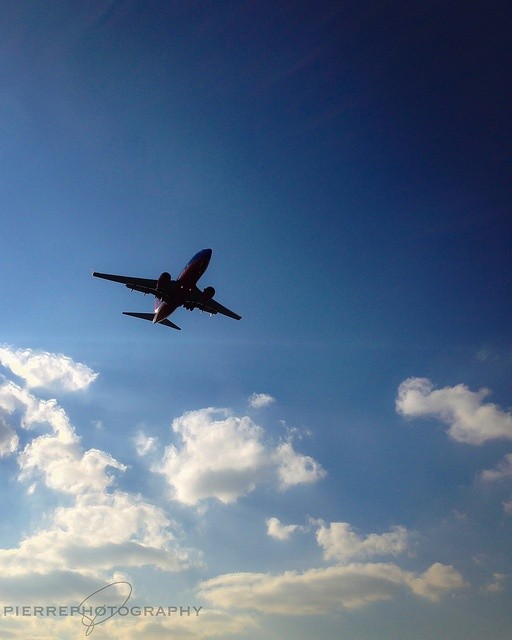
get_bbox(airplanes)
[92,248,241,330]
